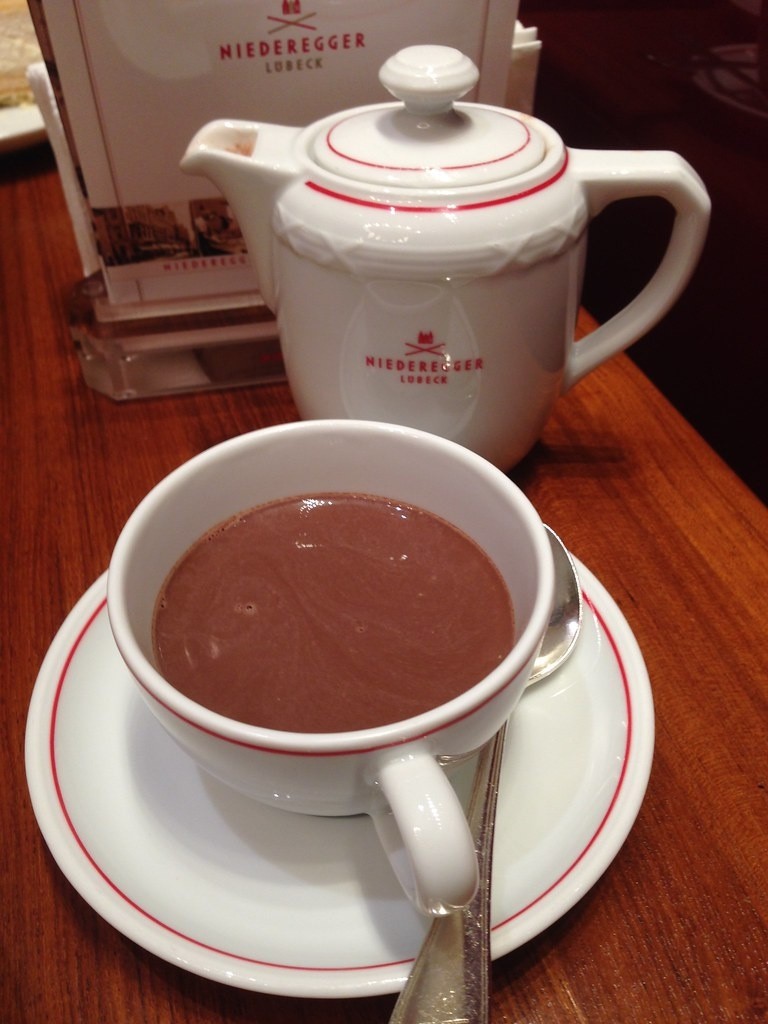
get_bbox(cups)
[110,421,557,912]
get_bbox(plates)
[27,569,656,997]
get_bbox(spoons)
[390,529,619,1024]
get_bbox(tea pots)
[186,46,711,472]
